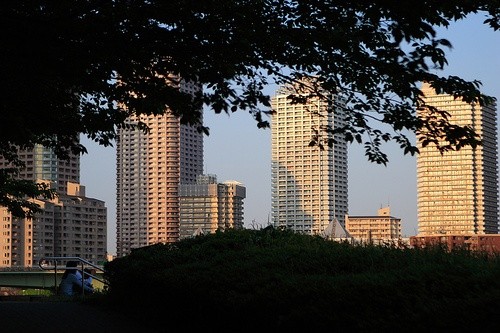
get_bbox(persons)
[76,268,94,288]
[60,260,93,298]
